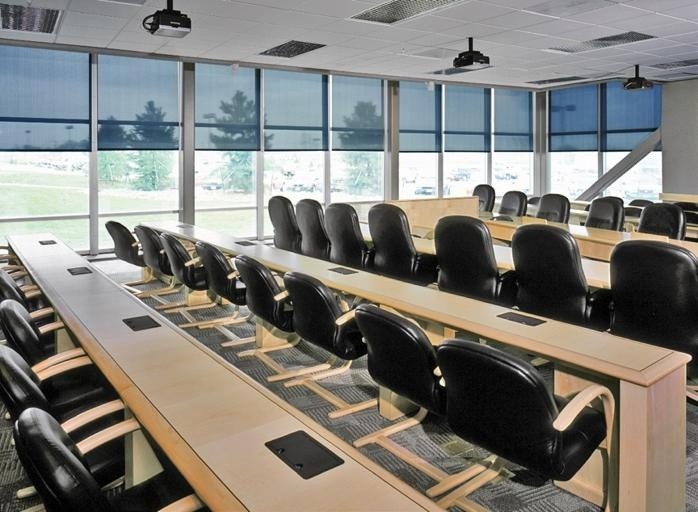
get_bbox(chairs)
[233,251,334,383]
[510,222,611,332]
[0,345,114,447]
[283,269,381,420]
[603,241,696,401]
[266,193,303,252]
[352,301,497,498]
[160,229,241,328]
[321,200,371,270]
[434,212,516,303]
[366,203,432,283]
[0,243,48,313]
[472,184,698,236]
[134,223,186,309]
[295,198,330,260]
[104,219,177,296]
[438,332,619,511]
[193,241,258,348]
[0,299,87,372]
[9,406,203,511]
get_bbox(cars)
[443,167,473,181]
[200,172,233,190]
[413,181,451,196]
[261,168,349,193]
[492,165,518,180]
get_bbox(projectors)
[623,77,653,91]
[141,10,191,39]
[452,51,491,72]
